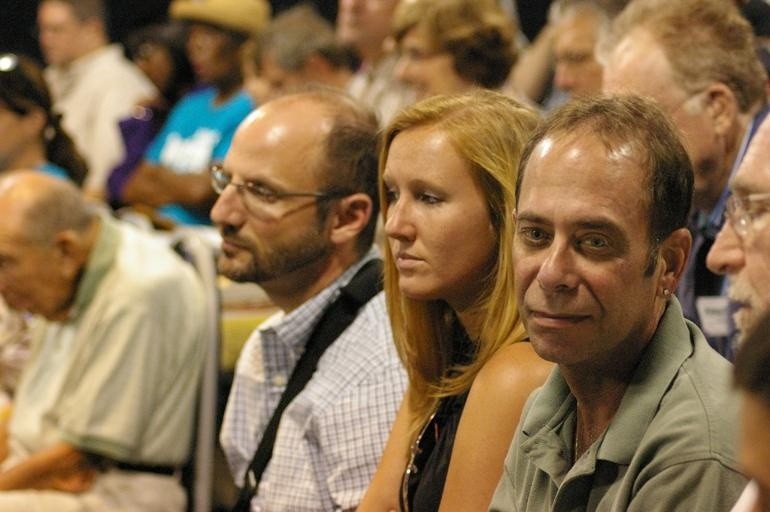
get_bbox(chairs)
[165,230,220,512]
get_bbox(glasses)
[206,160,350,214]
[722,191,770,235]
